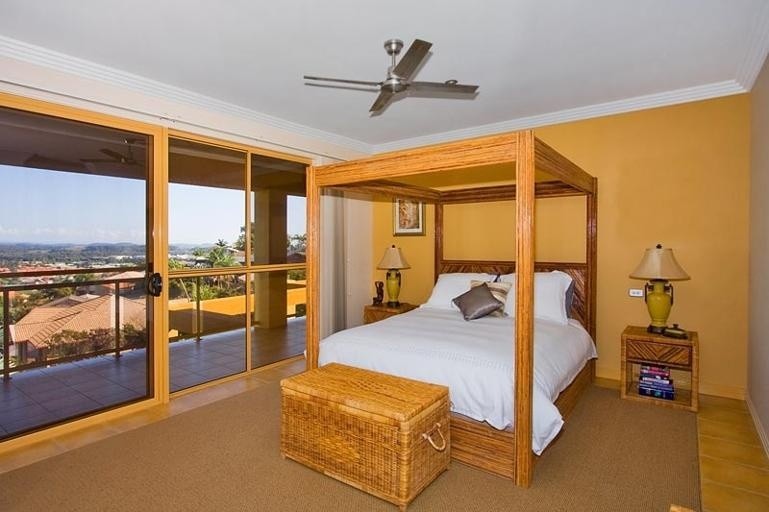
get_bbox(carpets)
[3,369,701,512]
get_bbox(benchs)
[279,360,452,512]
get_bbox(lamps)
[375,243,411,306]
[630,240,691,334]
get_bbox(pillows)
[498,268,573,327]
[468,279,513,319]
[454,284,502,320]
[424,270,497,311]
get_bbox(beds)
[306,257,602,486]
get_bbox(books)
[639,364,674,400]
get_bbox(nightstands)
[617,323,700,414]
[363,302,417,324]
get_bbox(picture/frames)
[390,198,427,238]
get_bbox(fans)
[298,34,479,116]
[82,135,149,171]
